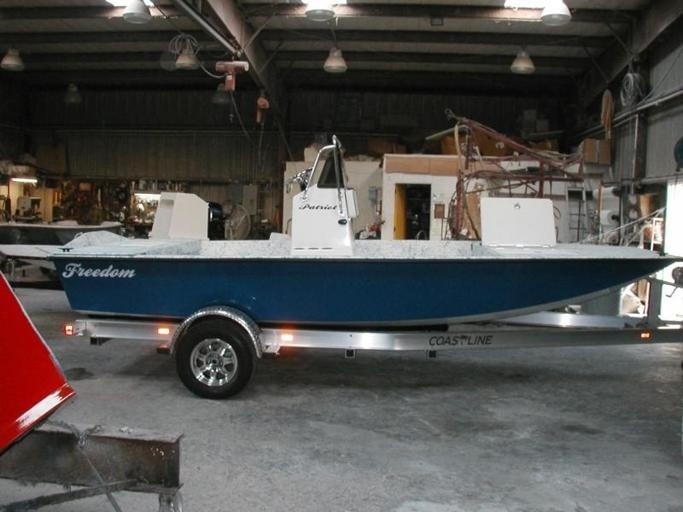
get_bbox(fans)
[221,204,251,240]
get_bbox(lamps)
[506,41,540,77]
[537,1,576,31]
[301,3,341,23]
[322,46,352,77]
[172,40,201,74]
[119,1,156,25]
[0,46,27,72]
[62,82,86,106]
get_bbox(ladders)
[563,156,588,243]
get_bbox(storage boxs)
[580,140,612,166]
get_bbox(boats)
[0,218,123,272]
[43,129,683,330]
